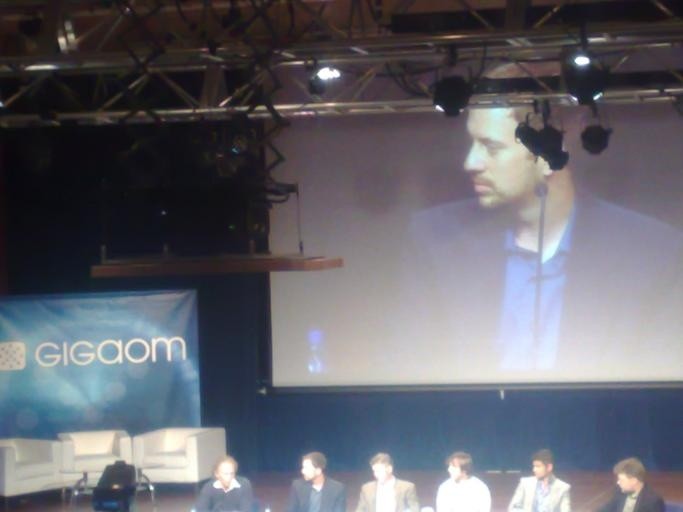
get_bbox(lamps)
[422,43,615,173]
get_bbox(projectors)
[92,461,136,512]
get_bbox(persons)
[354,451,419,512]
[507,448,572,511]
[590,456,664,511]
[194,454,252,512]
[385,62,682,384]
[286,451,345,511]
[434,450,491,511]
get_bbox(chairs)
[0,425,228,512]
